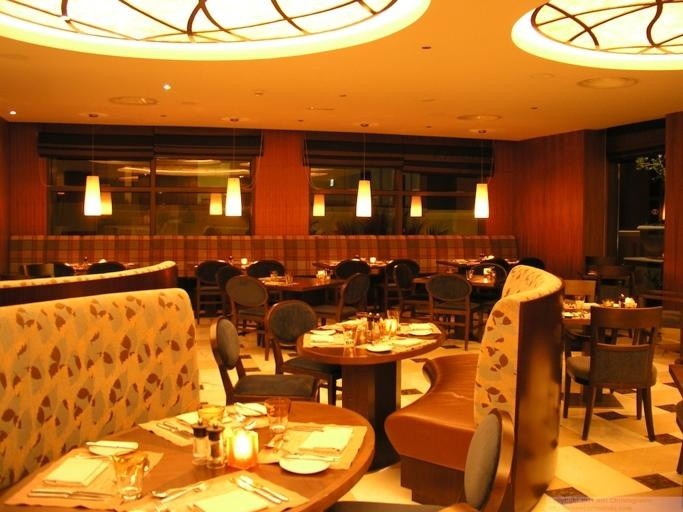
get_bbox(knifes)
[27,488,114,503]
[226,472,292,506]
[282,455,339,463]
[155,421,192,441]
[86,442,134,452]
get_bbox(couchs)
[384,264,566,510]
[0,288,227,512]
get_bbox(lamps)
[473,127,489,219]
[409,194,423,218]
[99,183,112,215]
[354,123,374,217]
[224,117,241,217]
[208,192,223,216]
[83,112,102,217]
[312,194,326,218]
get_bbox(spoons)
[148,484,196,498]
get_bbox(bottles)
[228,256,233,265]
[82,256,88,270]
[188,420,226,470]
[400,323,410,335]
[330,269,337,280]
[620,294,625,309]
[270,271,277,282]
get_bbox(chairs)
[246,257,682,361]
[3,257,177,306]
[224,272,276,360]
[194,257,235,325]
[265,299,345,407]
[209,315,320,408]
[564,306,657,444]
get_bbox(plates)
[367,345,393,352]
[176,401,267,433]
[308,336,333,342]
[278,454,332,476]
[309,329,336,336]
[88,441,138,455]
[411,330,431,337]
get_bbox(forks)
[158,480,212,502]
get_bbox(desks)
[297,315,447,473]
[0,396,376,512]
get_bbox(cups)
[574,294,584,311]
[113,450,146,502]
[263,396,293,433]
[466,269,473,281]
[342,312,398,347]
[315,271,327,284]
[284,272,294,285]
[368,256,376,264]
